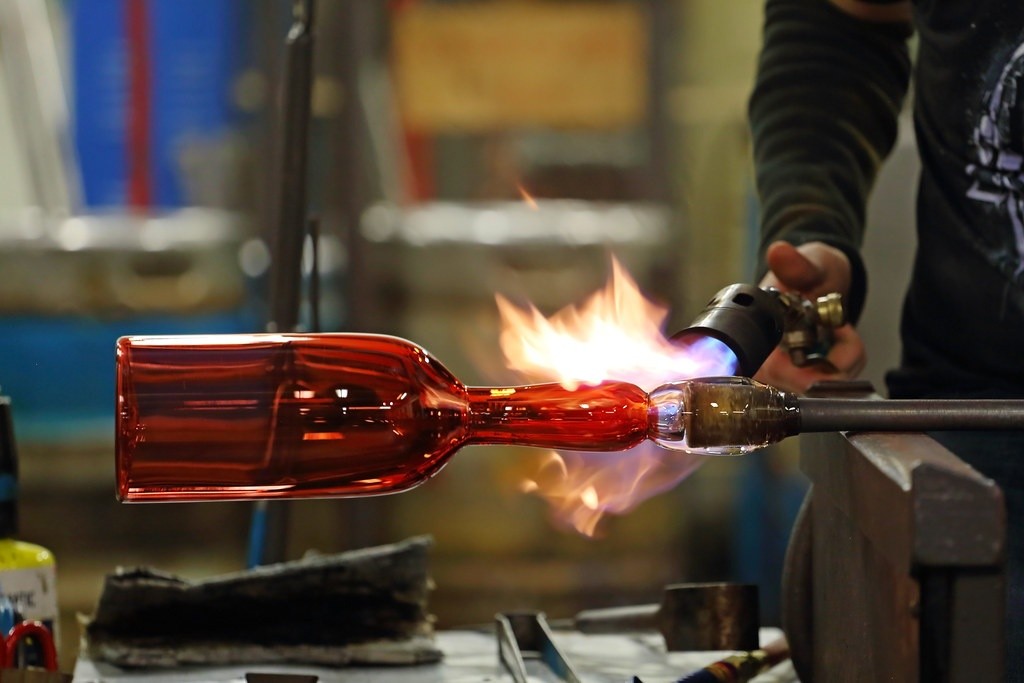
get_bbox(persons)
[746,0,1024,683]
